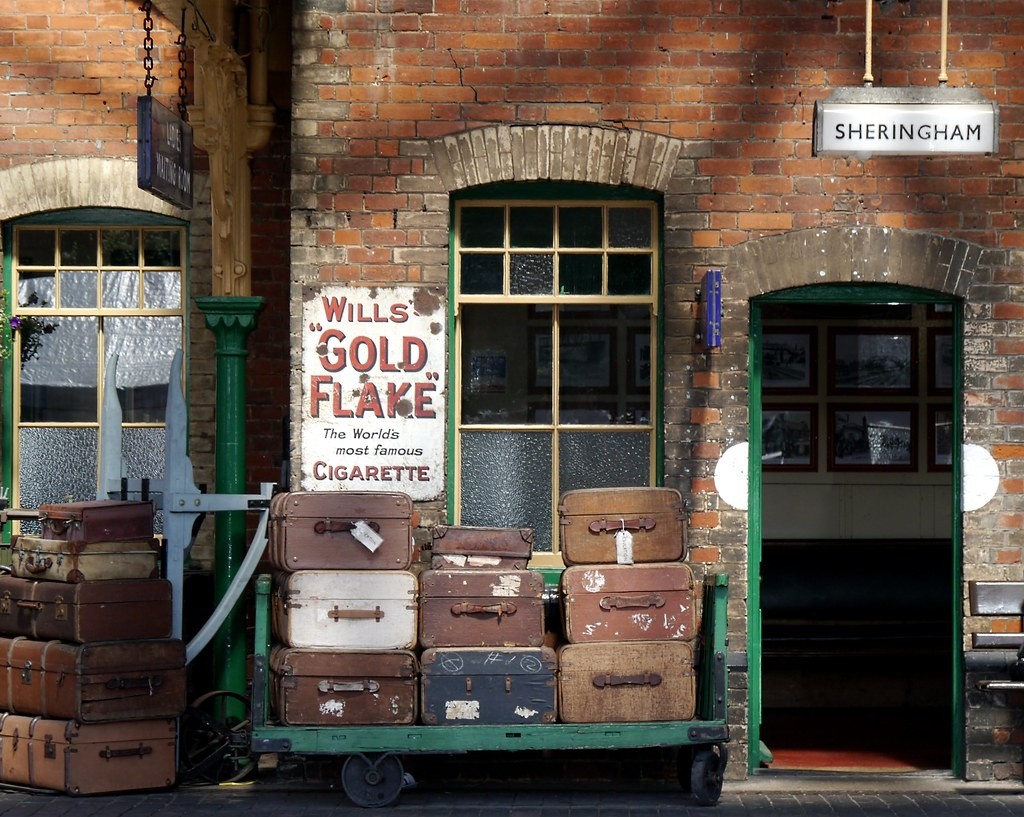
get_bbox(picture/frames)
[927,327,954,397]
[826,325,919,395]
[763,403,819,471]
[527,326,617,395]
[926,402,954,472]
[527,401,618,424]
[527,304,618,319]
[626,326,650,394]
[763,325,818,395]
[927,304,954,320]
[826,402,919,472]
[626,401,650,425]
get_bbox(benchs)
[759,538,953,709]
[969,580,1024,691]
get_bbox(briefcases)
[431,524,533,569]
[559,564,697,642]
[269,488,418,571]
[419,569,545,646]
[273,570,419,652]
[269,645,420,727]
[39,499,153,541]
[0,712,182,797]
[557,487,687,569]
[557,643,697,723]
[0,575,174,645]
[11,533,157,583]
[0,637,186,723]
[420,647,557,724]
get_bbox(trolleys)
[242,565,732,811]
